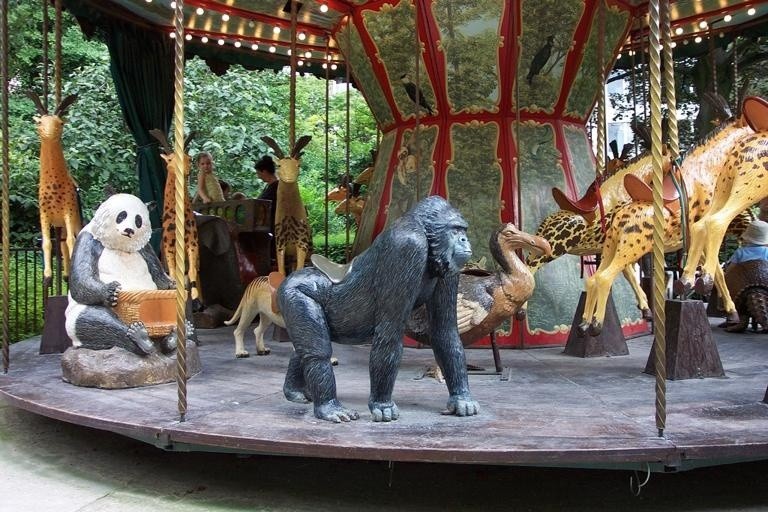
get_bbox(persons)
[194,150,226,205]
[220,180,230,199]
[253,154,280,265]
[727,219,768,262]
[230,191,247,199]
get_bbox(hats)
[741,220,768,245]
